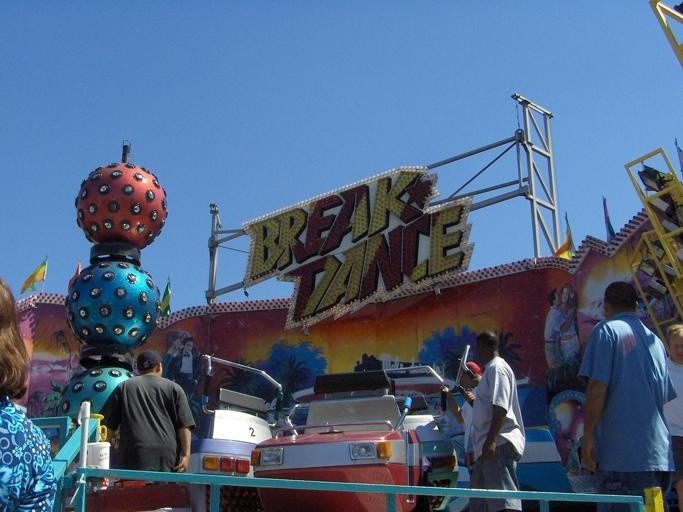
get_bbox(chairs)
[305,370,405,433]
[203,387,269,418]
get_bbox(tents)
[69,263,79,291]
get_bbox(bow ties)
[184,353,190,357]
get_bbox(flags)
[159,282,171,316]
[555,217,574,259]
[20,260,47,295]
[604,198,616,241]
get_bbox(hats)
[138,349,163,370]
[463,362,482,376]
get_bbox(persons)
[660,322,682,512]
[543,287,576,369]
[442,359,482,478]
[163,336,182,379]
[0,279,57,511]
[95,347,197,484]
[560,283,579,360]
[466,331,525,511]
[580,281,676,511]
[169,337,202,399]
